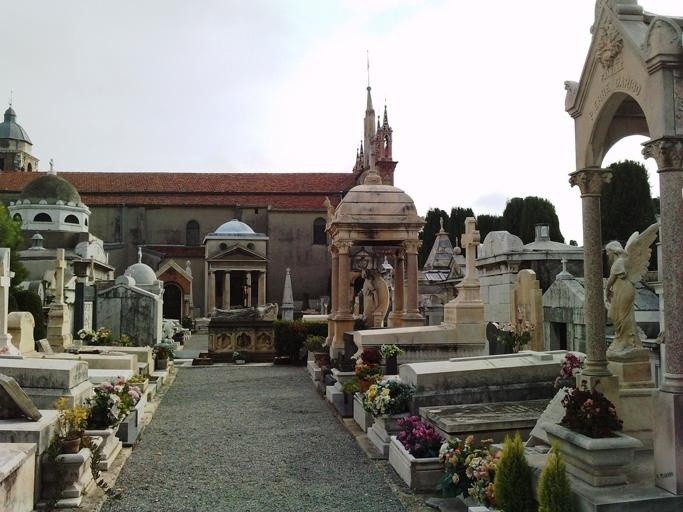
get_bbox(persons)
[604,222,659,352]
